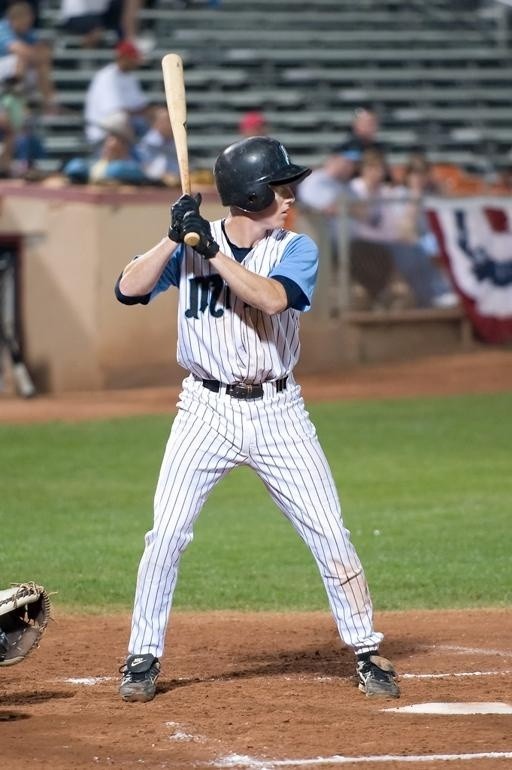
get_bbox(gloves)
[178,215,219,260]
[168,192,201,243]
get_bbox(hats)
[114,42,138,61]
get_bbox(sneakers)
[118,653,160,703]
[355,655,401,700]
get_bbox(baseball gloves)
[0,581,58,666]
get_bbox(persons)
[297,102,460,311]
[114,134,400,701]
[240,110,267,139]
[0,0,186,191]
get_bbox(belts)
[203,376,288,399]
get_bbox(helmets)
[213,136,312,215]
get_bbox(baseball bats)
[162,53,200,247]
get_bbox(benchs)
[24,4,511,187]
